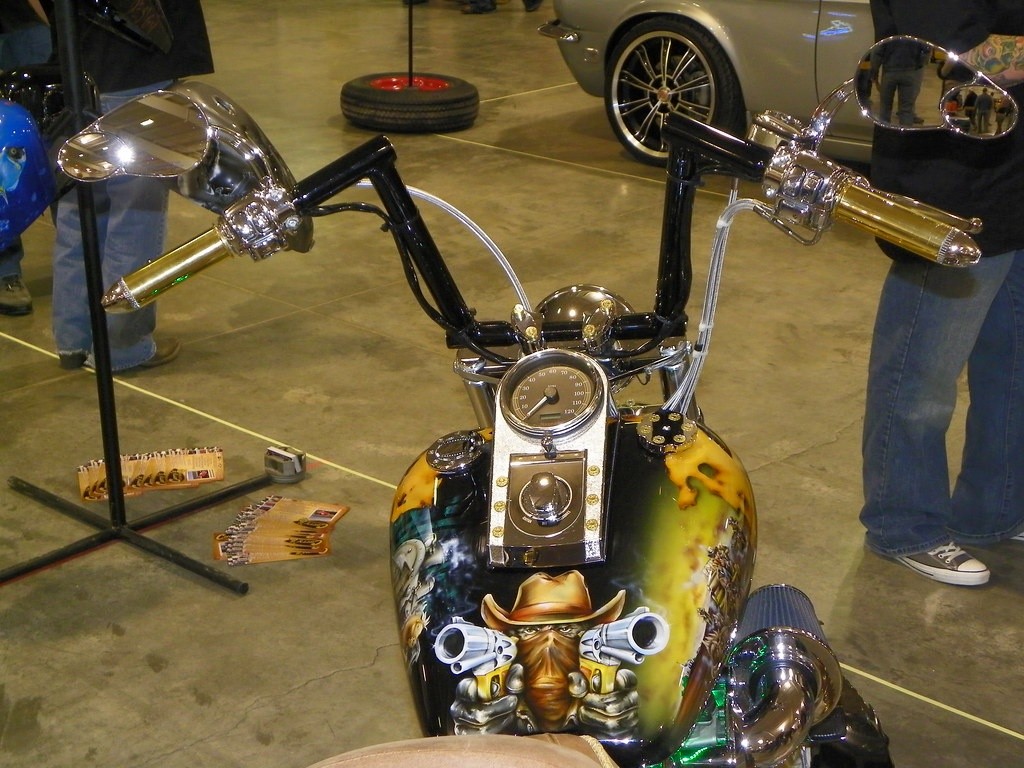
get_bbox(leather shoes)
[139,336,182,364]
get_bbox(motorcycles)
[57,5,1019,767]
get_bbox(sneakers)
[0,275,34,317]
[886,542,990,587]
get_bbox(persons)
[856,0,1024,585]
[43,1,214,374]
[850,40,1017,138]
[1,0,54,314]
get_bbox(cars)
[532,2,898,183]
